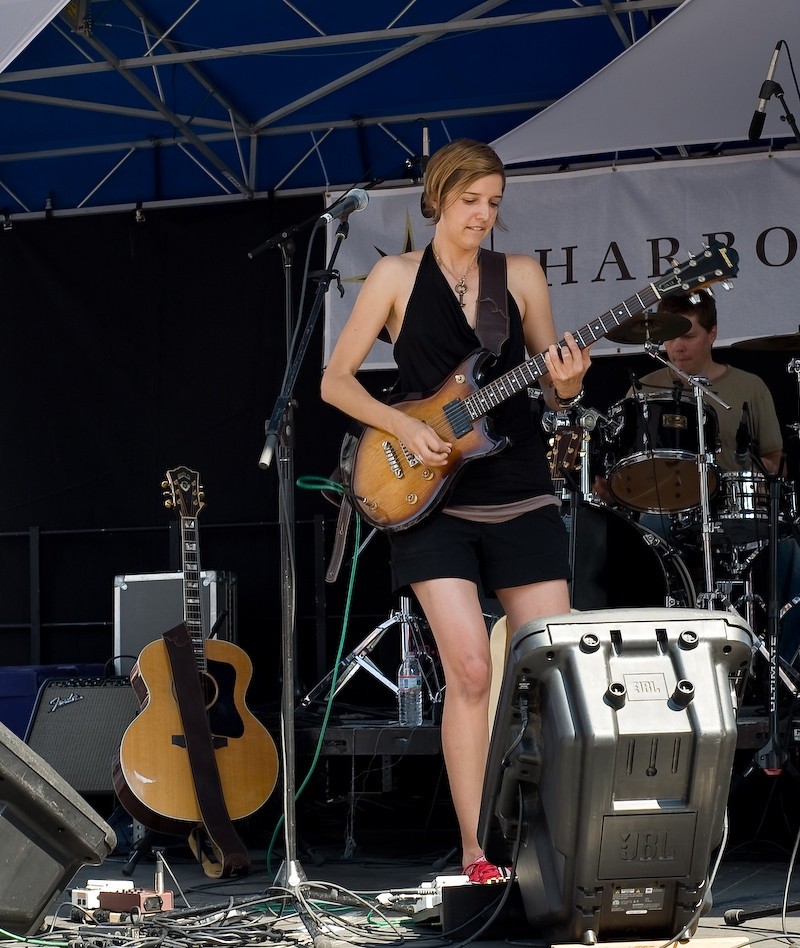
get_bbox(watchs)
[553,386,586,408]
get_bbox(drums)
[607,390,719,515]
[561,521,698,611]
[709,470,796,542]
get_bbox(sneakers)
[462,861,502,884]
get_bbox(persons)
[320,139,592,886]
[596,290,800,721]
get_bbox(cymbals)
[604,311,693,345]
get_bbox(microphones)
[316,188,369,227]
[422,126,430,173]
[748,42,783,143]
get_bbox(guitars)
[487,427,590,741]
[349,241,741,534]
[111,466,279,832]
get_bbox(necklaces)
[432,240,480,308]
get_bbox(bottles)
[398,652,422,727]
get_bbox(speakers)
[0,720,117,937]
[22,677,147,821]
[474,608,759,945]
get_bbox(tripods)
[154,225,419,948]
[295,594,445,728]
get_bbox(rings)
[414,453,423,462]
